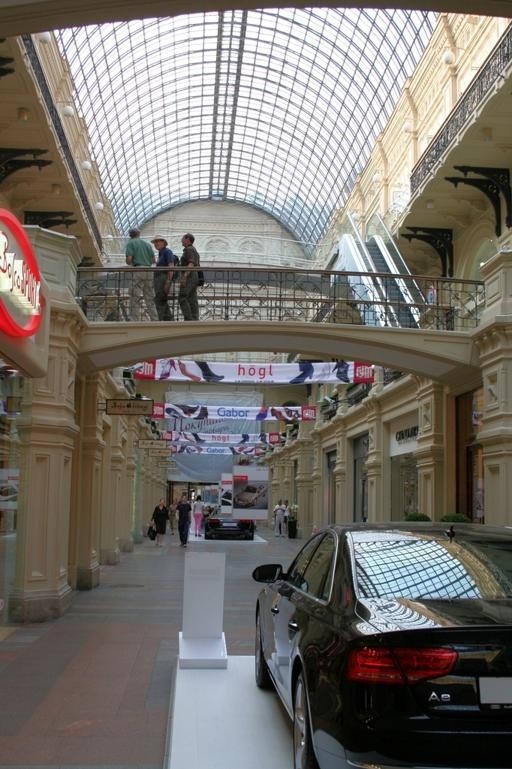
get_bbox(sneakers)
[203,371,224,379]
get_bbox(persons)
[77,255,96,318]
[283,500,290,535]
[176,233,201,322]
[125,228,161,322]
[273,499,286,537]
[149,233,176,322]
[192,494,206,538]
[168,499,178,536]
[151,497,169,548]
[176,495,192,548]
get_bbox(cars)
[252,520,511,769]
[204,507,255,540]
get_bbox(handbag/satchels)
[198,271,204,286]
[148,526,157,540]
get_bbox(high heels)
[332,360,350,382]
[160,359,176,379]
[289,367,314,384]
[172,432,269,454]
[164,405,302,422]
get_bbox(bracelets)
[166,279,172,283]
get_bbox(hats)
[150,234,168,246]
